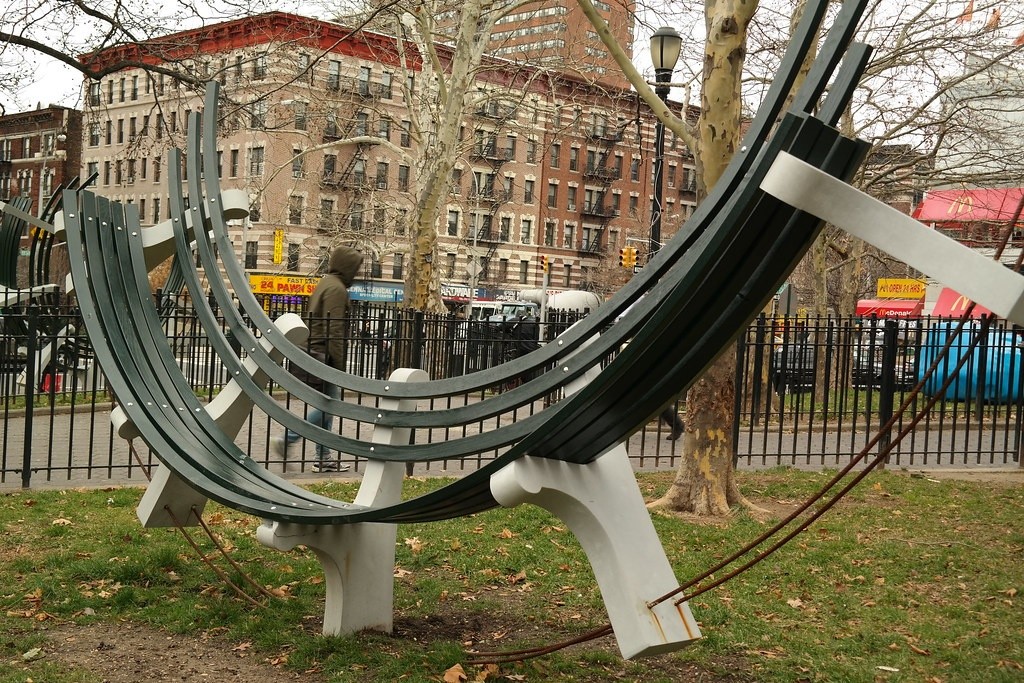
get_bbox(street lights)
[645,23,684,268]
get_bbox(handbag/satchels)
[288,345,331,387]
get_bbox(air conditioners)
[613,209,621,216]
[294,170,303,178]
[523,238,530,244]
[378,183,387,189]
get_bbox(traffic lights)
[619,248,631,269]
[630,247,639,267]
[541,255,549,274]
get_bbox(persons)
[272,244,364,472]
[616,264,686,439]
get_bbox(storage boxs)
[569,204,576,210]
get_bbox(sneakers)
[312,455,350,472]
[270,436,299,461]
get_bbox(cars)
[853,352,872,386]
[868,344,915,387]
[771,344,815,387]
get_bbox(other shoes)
[666,420,686,440]
